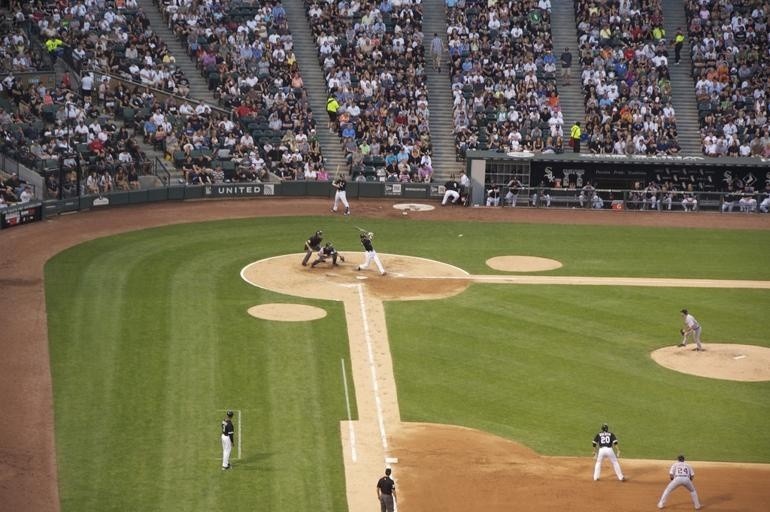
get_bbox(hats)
[385,468,391,473]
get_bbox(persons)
[1,0,770,223]
[590,423,625,480]
[356,230,387,276]
[220,410,236,469]
[658,453,704,509]
[300,230,323,265]
[310,241,345,269]
[678,308,704,351]
[375,468,398,512]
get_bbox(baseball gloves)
[680,328,684,336]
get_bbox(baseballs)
[459,234,463,236]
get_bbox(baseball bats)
[355,226,371,234]
[333,165,340,184]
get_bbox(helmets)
[359,233,368,238]
[340,173,346,178]
[680,309,688,314]
[326,241,332,248]
[316,230,323,236]
[227,410,234,417]
[601,423,609,431]
